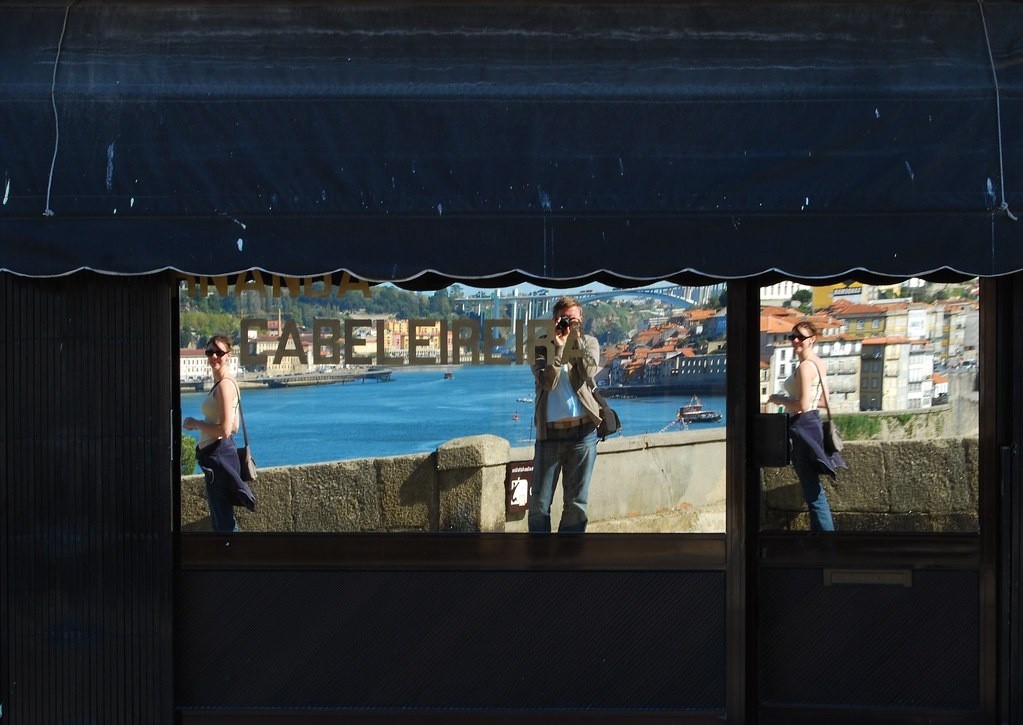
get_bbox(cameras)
[559,316,573,329]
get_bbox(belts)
[546,416,591,429]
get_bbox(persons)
[766,321,848,530]
[184,336,256,532]
[527,298,600,532]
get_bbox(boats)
[677,393,722,423]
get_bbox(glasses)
[788,334,813,342]
[205,350,230,357]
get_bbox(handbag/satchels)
[822,420,844,457]
[236,446,257,481]
[597,409,621,438]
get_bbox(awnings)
[0,1,1023,291]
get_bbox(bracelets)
[193,421,199,429]
